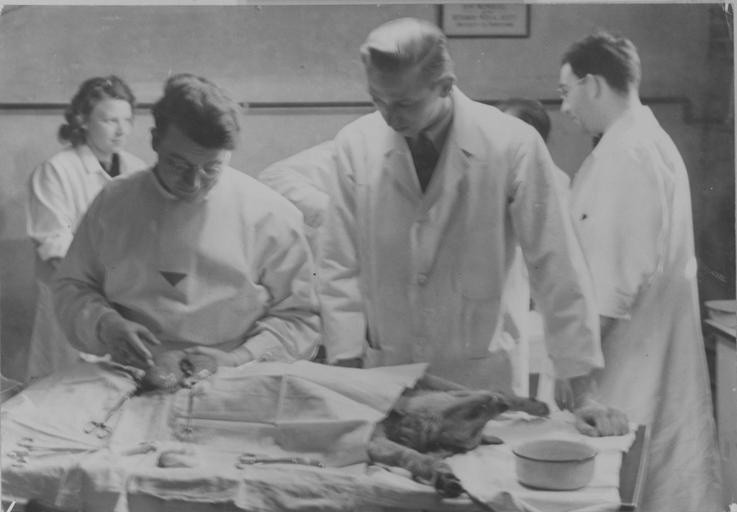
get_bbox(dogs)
[110,345,551,500]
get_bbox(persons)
[540,31,723,510]
[308,16,630,438]
[495,98,570,400]
[53,71,322,392]
[18,74,149,392]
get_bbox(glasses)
[159,147,221,179]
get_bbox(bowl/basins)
[704,298,736,327]
[510,437,600,492]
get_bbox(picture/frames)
[435,4,535,39]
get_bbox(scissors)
[84,397,125,439]
[237,451,321,469]
